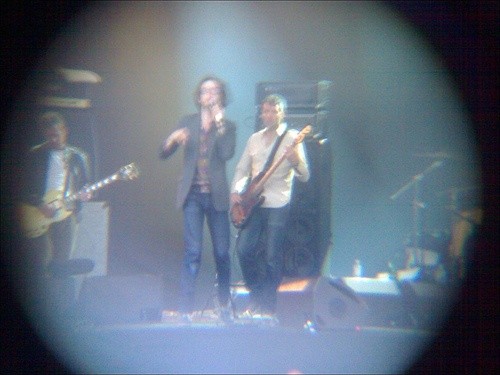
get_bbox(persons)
[229,94,311,327]
[16,111,94,277]
[157,76,237,327]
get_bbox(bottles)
[353,259,361,277]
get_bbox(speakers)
[340,277,453,328]
[253,79,333,277]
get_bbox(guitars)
[230,124,310,229]
[17,162,140,238]
